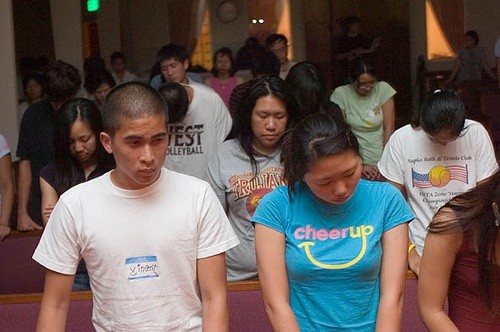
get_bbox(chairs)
[1,230,448,332]
[435,69,500,148]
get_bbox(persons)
[0,33,398,295]
[444,30,500,84]
[334,15,383,83]
[249,111,417,332]
[32,81,241,332]
[418,167,500,332]
[378,88,500,276]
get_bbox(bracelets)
[409,244,416,252]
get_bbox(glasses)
[357,80,376,89]
